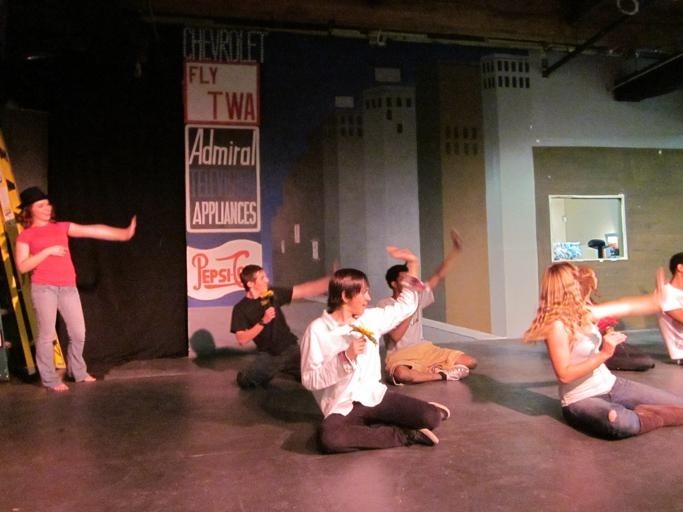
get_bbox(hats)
[17,186,49,208]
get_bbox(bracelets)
[258,319,268,327]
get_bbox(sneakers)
[405,428,439,446]
[437,365,469,381]
[428,402,450,421]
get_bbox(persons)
[300,245,451,453]
[230,253,339,391]
[521,252,683,440]
[14,186,138,392]
[376,227,478,385]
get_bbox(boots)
[634,404,683,433]
